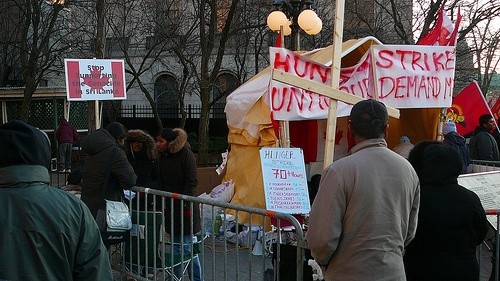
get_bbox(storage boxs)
[220,213,235,237]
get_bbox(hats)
[351,99,388,123]
[443,121,457,135]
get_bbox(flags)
[446,81,492,137]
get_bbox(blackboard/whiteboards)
[258,147,312,215]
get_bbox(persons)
[1,120,114,281]
[56,119,79,173]
[404,140,489,281]
[307,99,421,281]
[443,121,470,175]
[471,114,500,169]
[79,122,203,281]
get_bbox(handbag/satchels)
[105,201,132,233]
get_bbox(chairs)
[107,205,210,281]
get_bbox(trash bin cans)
[125,211,163,278]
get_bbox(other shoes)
[66,169,71,172]
[59,168,65,172]
